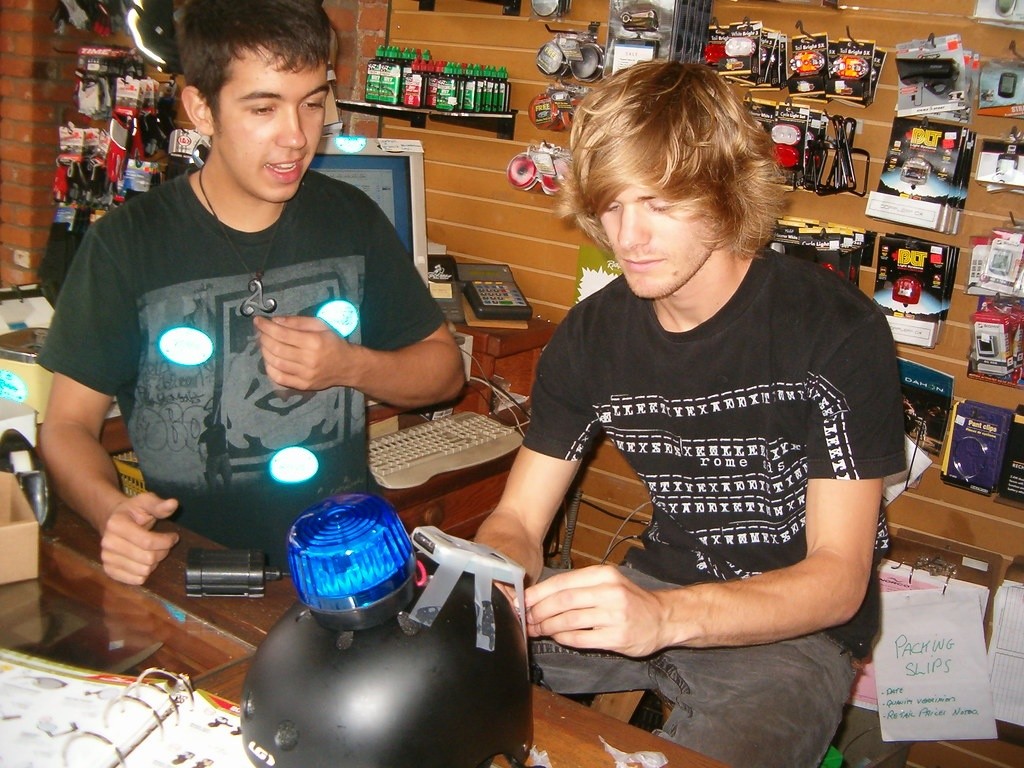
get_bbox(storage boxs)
[0,470,40,585]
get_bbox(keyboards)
[366,411,524,489]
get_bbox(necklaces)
[199,158,290,316]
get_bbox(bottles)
[365,45,511,114]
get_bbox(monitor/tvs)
[307,136,429,295]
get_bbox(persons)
[474,60,908,768]
[33,0,466,586]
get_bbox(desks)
[0,315,733,768]
